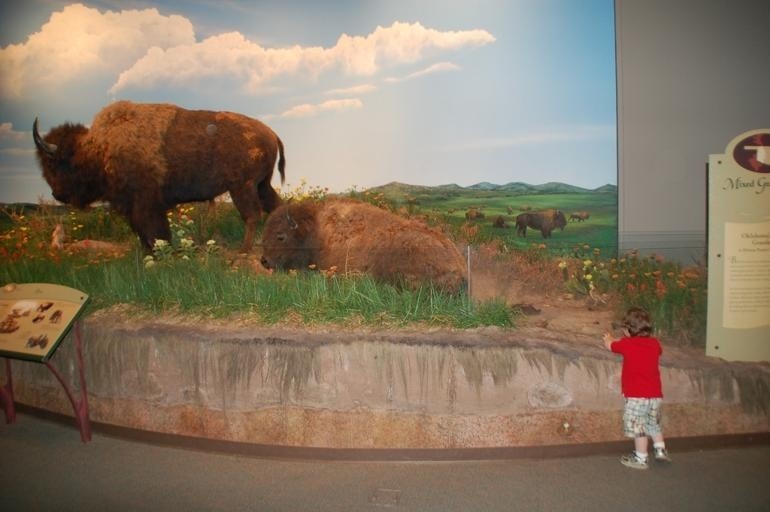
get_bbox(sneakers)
[654,447,675,466]
[620,452,650,470]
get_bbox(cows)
[31,100,288,261]
[259,197,472,297]
[446,204,590,241]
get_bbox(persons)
[602,307,672,470]
